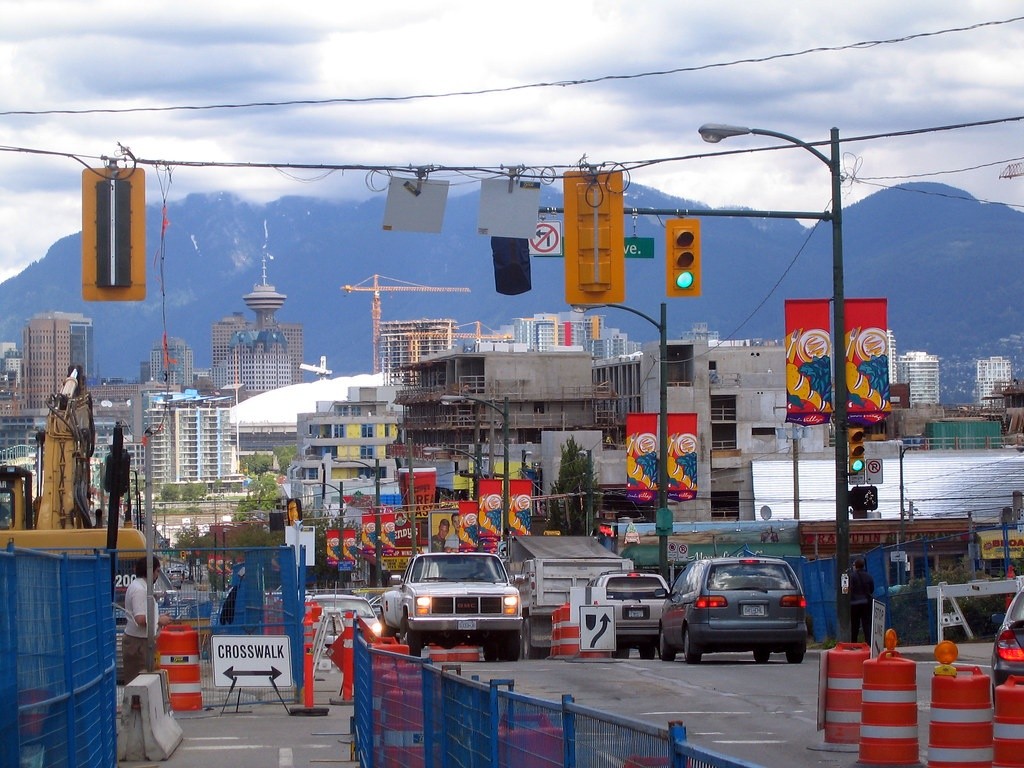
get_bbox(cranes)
[405,321,514,353]
[339,273,473,375]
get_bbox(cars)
[304,593,383,640]
[989,585,1024,706]
[652,554,809,665]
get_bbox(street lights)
[303,481,344,587]
[422,445,484,501]
[697,122,852,647]
[438,393,511,543]
[332,458,382,588]
[899,444,921,543]
[568,301,671,586]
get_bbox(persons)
[444,514,459,552]
[122,557,173,686]
[432,518,450,552]
[849,558,874,646]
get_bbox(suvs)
[378,550,527,663]
[587,569,672,659]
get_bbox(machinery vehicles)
[0,364,174,624]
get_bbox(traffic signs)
[210,634,294,687]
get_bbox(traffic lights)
[666,218,701,298]
[849,426,866,472]
[852,486,879,511]
